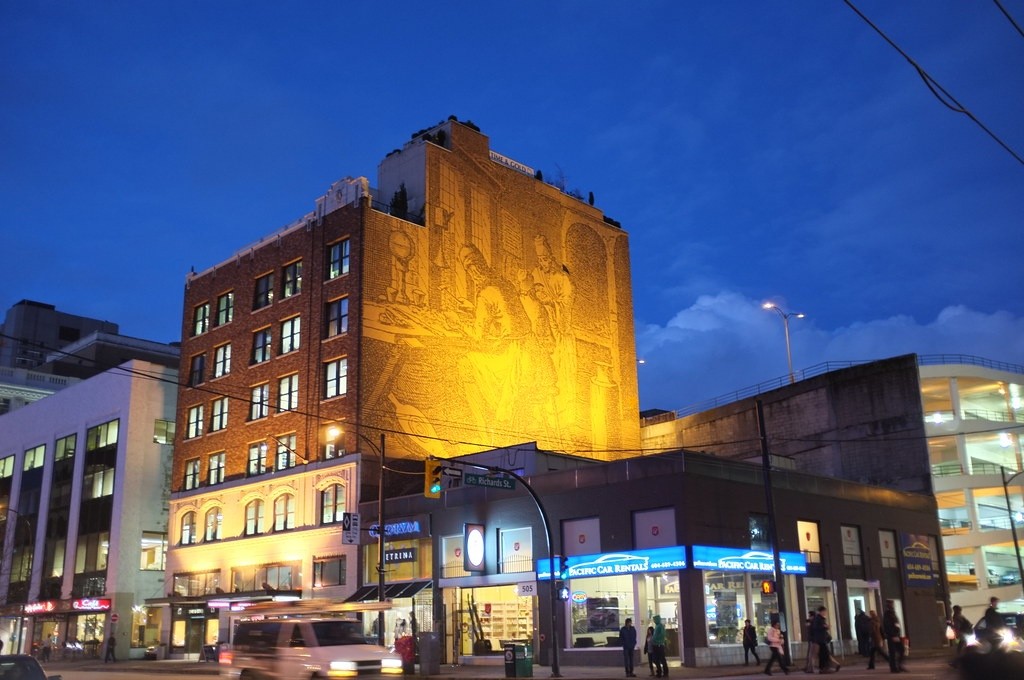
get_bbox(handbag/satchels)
[880,624,887,640]
[764,636,771,645]
[900,637,909,657]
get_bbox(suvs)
[219,612,404,680]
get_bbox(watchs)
[984,596,1003,643]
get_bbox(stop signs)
[110,613,118,623]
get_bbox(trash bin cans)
[505,636,533,678]
[418,631,440,677]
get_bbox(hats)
[991,597,1000,601]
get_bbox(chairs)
[203,645,218,662]
[574,637,595,648]
[607,637,623,647]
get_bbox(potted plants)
[389,181,408,220]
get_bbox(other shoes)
[764,668,834,677]
[867,666,875,670]
[743,664,749,666]
[624,671,670,680]
[835,665,841,672]
[756,662,760,666]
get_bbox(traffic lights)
[763,580,776,594]
[555,580,569,600]
[425,459,445,499]
[560,556,568,578]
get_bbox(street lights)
[329,427,386,647]
[763,303,805,384]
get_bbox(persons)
[855,600,909,674]
[643,615,668,677]
[620,618,636,676]
[408,611,418,657]
[762,618,789,676]
[953,605,973,653]
[43,633,50,663]
[743,619,761,666]
[105,632,117,663]
[802,605,841,673]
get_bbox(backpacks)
[961,618,973,635]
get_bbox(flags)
[342,580,433,602]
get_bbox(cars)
[145,643,158,656]
[961,612,1024,679]
[0,654,65,680]
